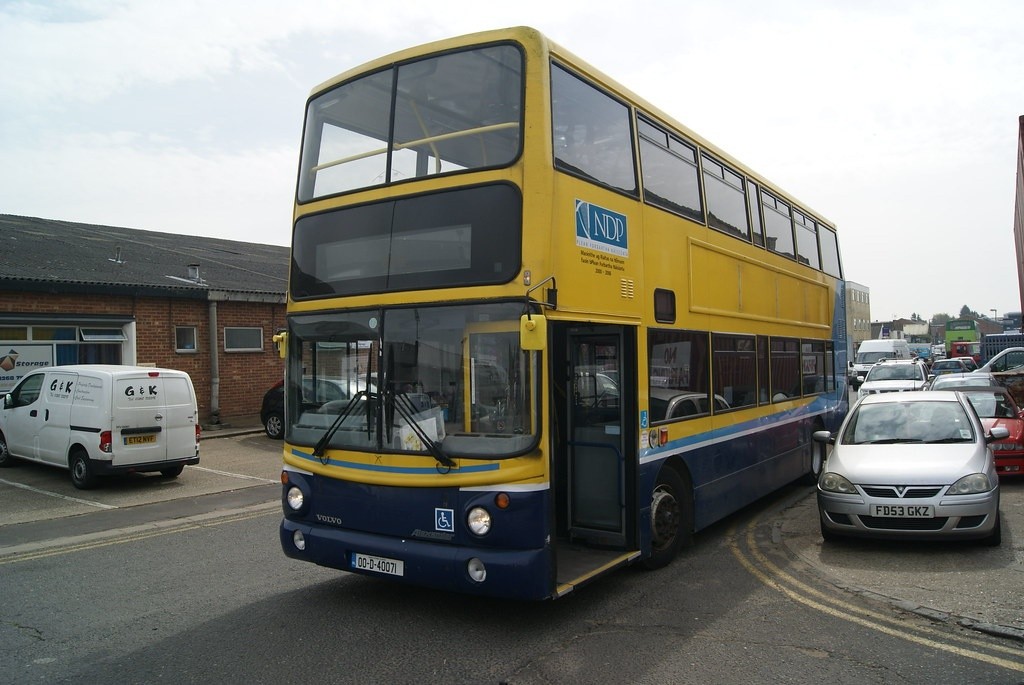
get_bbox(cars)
[932,347,946,359]
[928,373,1005,402]
[937,386,1024,481]
[812,391,1010,547]
[260,376,380,439]
[928,356,979,378]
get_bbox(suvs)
[857,358,932,399]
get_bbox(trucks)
[850,339,910,389]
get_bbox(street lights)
[991,309,997,322]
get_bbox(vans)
[910,347,931,362]
[0,364,199,488]
[971,346,1024,374]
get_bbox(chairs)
[929,406,961,437]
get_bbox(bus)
[274,27,848,605]
[945,321,984,359]
